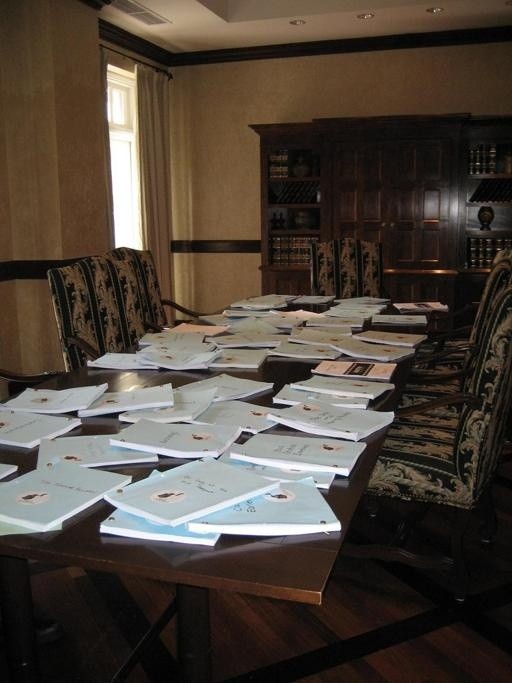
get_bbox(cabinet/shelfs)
[247,111,512,327]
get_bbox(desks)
[1,299,435,681]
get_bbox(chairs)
[398,281,511,417]
[412,260,511,376]
[44,245,206,375]
[361,307,512,604]
